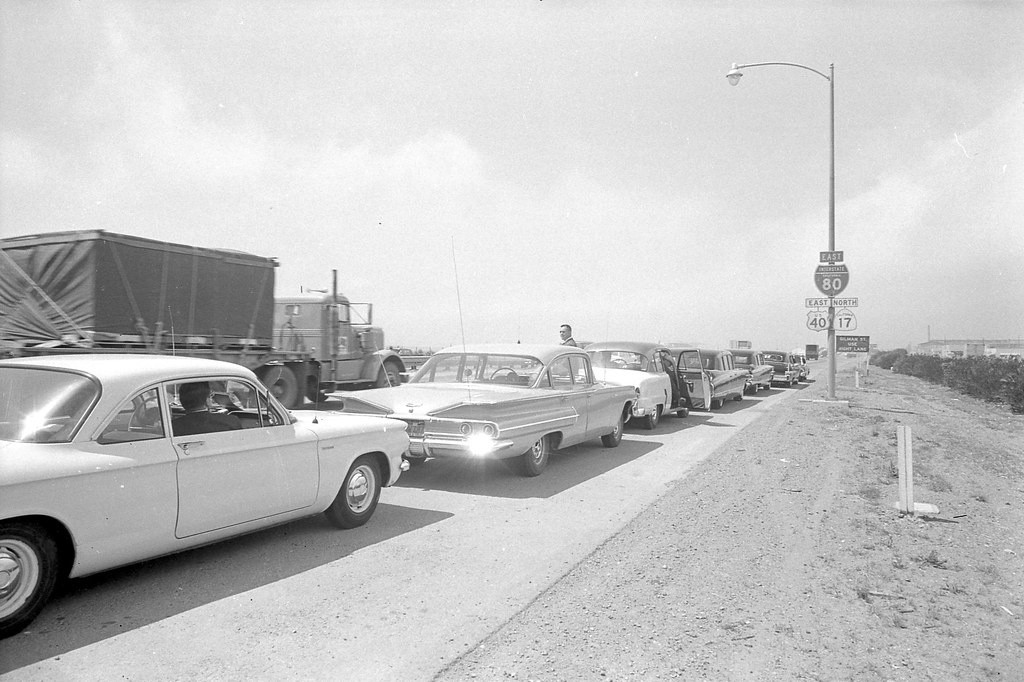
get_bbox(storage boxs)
[0,229,279,348]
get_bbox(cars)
[321,342,639,477]
[793,355,809,382]
[727,351,775,394]
[611,351,636,363]
[668,349,748,411]
[0,352,413,634]
[763,351,802,388]
[572,342,712,429]
[820,349,829,358]
[394,348,421,355]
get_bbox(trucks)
[805,344,820,360]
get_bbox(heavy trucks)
[0,228,408,410]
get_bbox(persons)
[172,382,241,438]
[662,354,686,403]
[559,324,576,347]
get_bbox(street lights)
[726,62,838,400]
